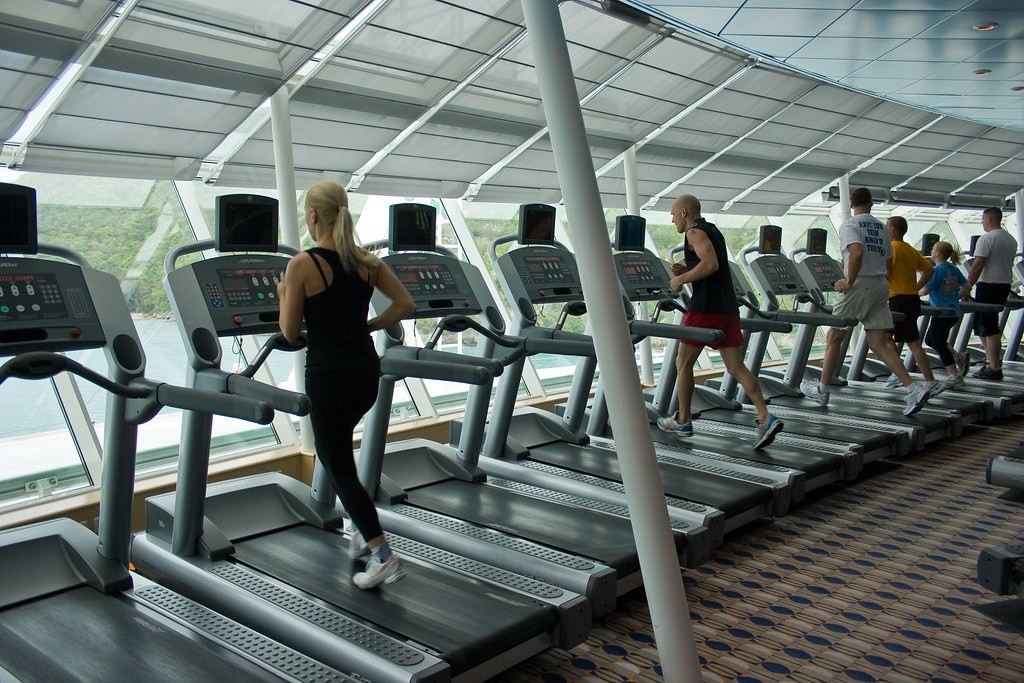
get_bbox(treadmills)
[0,183,1024,683]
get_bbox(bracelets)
[845,278,853,286]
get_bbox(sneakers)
[923,380,945,399]
[352,554,408,589]
[881,373,904,389]
[656,411,693,437]
[800,378,830,405]
[903,384,930,416]
[957,352,970,377]
[972,365,1004,380]
[943,375,967,390]
[752,413,784,449]
[978,358,1002,371]
[347,530,372,559]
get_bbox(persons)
[962,208,1018,383]
[882,215,945,400]
[276,181,416,588]
[655,193,783,449]
[917,242,970,386]
[800,188,932,416]
